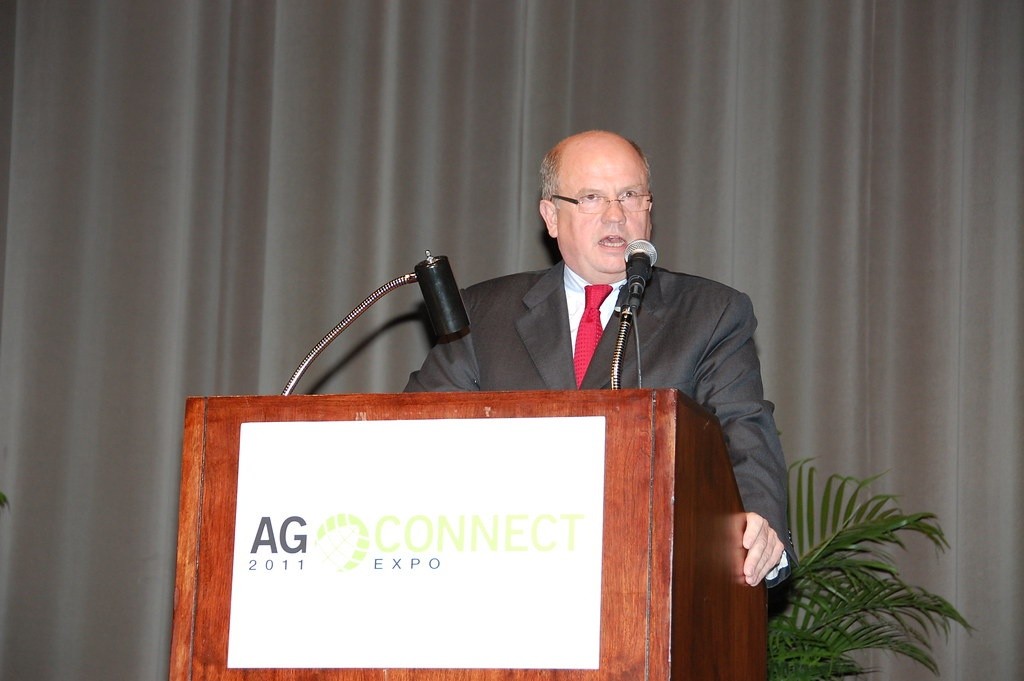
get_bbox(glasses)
[549,192,654,215]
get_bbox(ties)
[573,284,611,389]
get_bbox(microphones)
[625,238,658,311]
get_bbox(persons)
[404,129,792,587]
